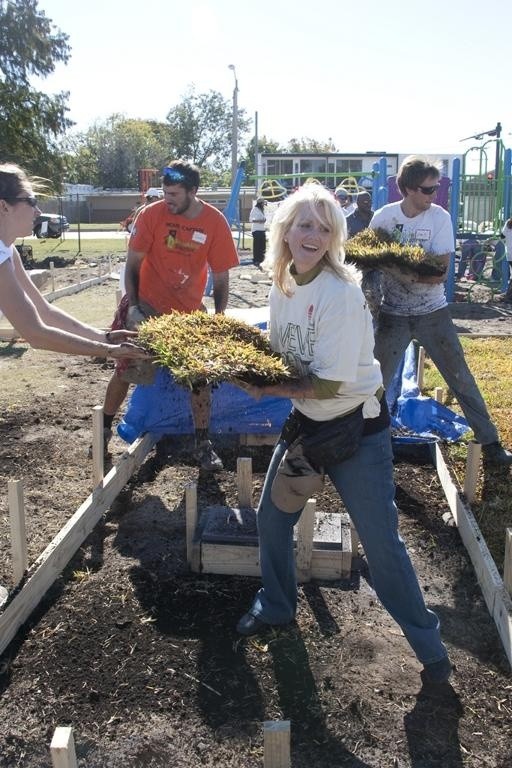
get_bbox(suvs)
[34,204,70,239]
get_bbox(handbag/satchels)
[302,412,364,469]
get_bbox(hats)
[148,186,158,197]
[270,434,326,514]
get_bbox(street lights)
[225,62,239,184]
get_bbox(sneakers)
[193,439,223,471]
[420,658,451,685]
[236,612,264,636]
[481,444,512,464]
[89,427,112,456]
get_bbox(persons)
[334,187,374,240]
[501,217,512,303]
[0,165,148,362]
[236,178,451,687]
[352,159,512,464]
[92,162,240,456]
[250,196,266,267]
[134,187,159,225]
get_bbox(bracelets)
[105,328,112,342]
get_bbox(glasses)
[6,197,37,206]
[162,167,187,183]
[418,183,440,194]
